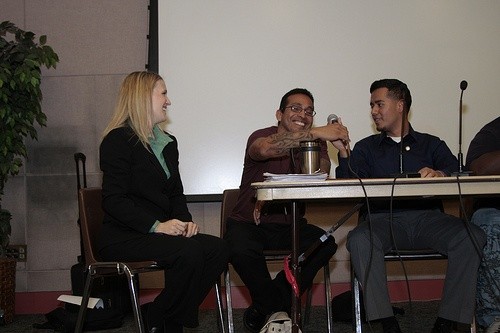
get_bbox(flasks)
[291,141,320,174]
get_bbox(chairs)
[219,187,449,333]
[75,187,225,333]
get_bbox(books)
[263,173,328,182]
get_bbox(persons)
[464,116,500,333]
[225,89,349,333]
[333,79,486,333]
[99,71,229,333]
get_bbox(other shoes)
[138,302,164,333]
[431,317,471,333]
[362,317,402,333]
[165,320,183,333]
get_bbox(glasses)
[284,105,316,117]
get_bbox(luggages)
[70,153,124,331]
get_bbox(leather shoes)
[242,302,266,333]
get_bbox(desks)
[250,174,500,333]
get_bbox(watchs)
[438,171,447,177]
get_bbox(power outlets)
[10,245,27,262]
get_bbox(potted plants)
[0,20,60,328]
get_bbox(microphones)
[451,80,475,178]
[391,83,422,177]
[327,114,348,143]
